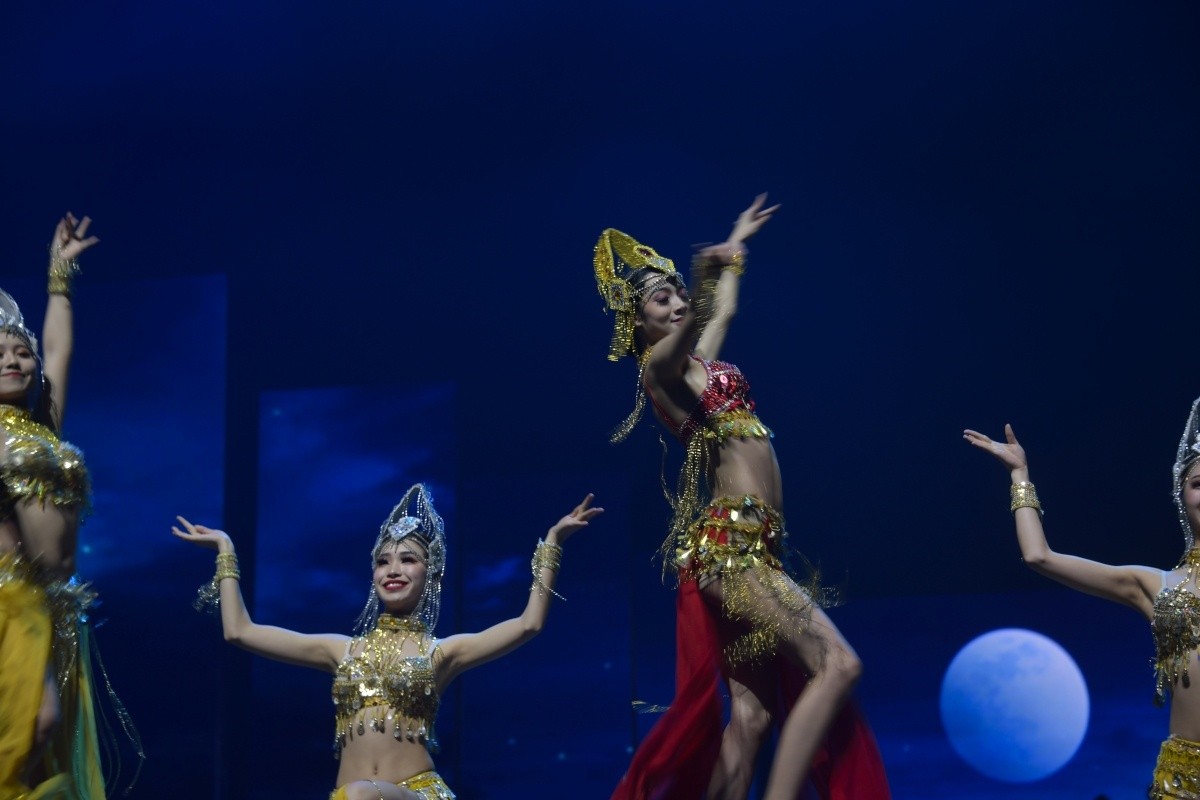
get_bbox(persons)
[171,481,603,799]
[0,209,156,800]
[964,398,1199,800]
[594,190,863,800]
